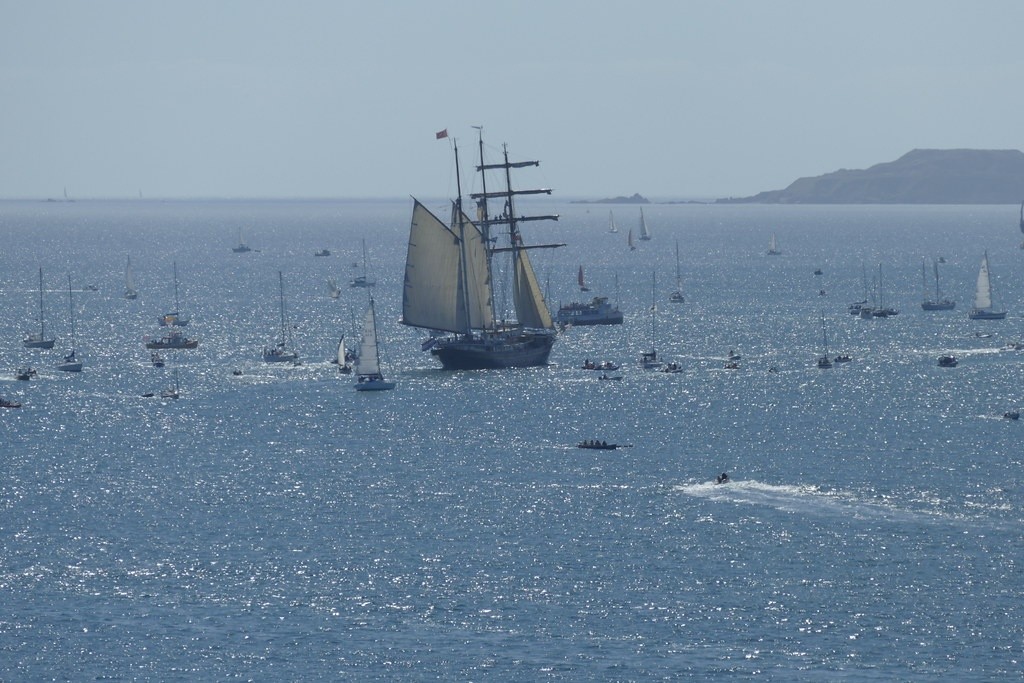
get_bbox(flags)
[436,130,447,139]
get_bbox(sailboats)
[969,250,1009,321]
[231,226,251,252]
[349,238,377,287]
[352,294,397,392]
[57,274,83,372]
[668,239,686,304]
[767,230,782,255]
[399,125,569,370]
[627,230,636,252]
[160,351,180,399]
[607,209,618,234]
[122,255,138,299]
[261,271,299,364]
[160,261,191,327]
[338,334,352,374]
[637,205,652,242]
[23,267,56,350]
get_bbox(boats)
[581,364,621,371]
[719,475,731,484]
[818,353,833,369]
[935,354,960,368]
[575,251,1024,383]
[575,443,617,450]
[0,396,21,408]
[16,369,36,381]
[315,249,331,257]
[145,329,198,350]
[599,376,623,381]
[556,295,625,327]
[835,355,853,363]
[1004,409,1020,420]
